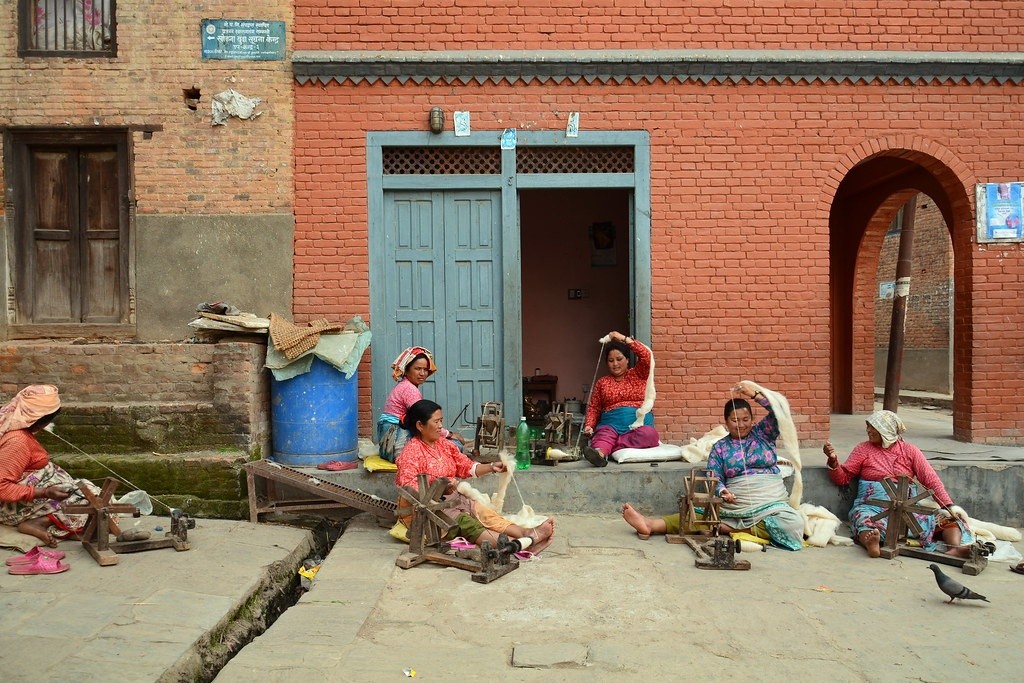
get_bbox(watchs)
[750,390,759,399]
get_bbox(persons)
[378,348,466,465]
[1,384,120,547]
[581,331,659,468]
[622,381,805,544]
[823,409,979,558]
[395,399,555,557]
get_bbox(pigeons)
[548,449,579,466]
[926,564,991,605]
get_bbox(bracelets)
[447,431,453,438]
[623,336,627,343]
[490,462,496,474]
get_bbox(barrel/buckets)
[561,399,582,412]
[515,417,530,471]
[531,429,536,439]
[270,355,358,467]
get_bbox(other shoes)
[583,446,608,467]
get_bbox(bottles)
[535,368,540,375]
[542,431,545,439]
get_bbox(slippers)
[1009,563,1024,574]
[447,536,478,550]
[513,550,534,561]
[327,461,358,470]
[638,529,650,540]
[8,554,70,575]
[317,460,337,469]
[6,544,66,565]
